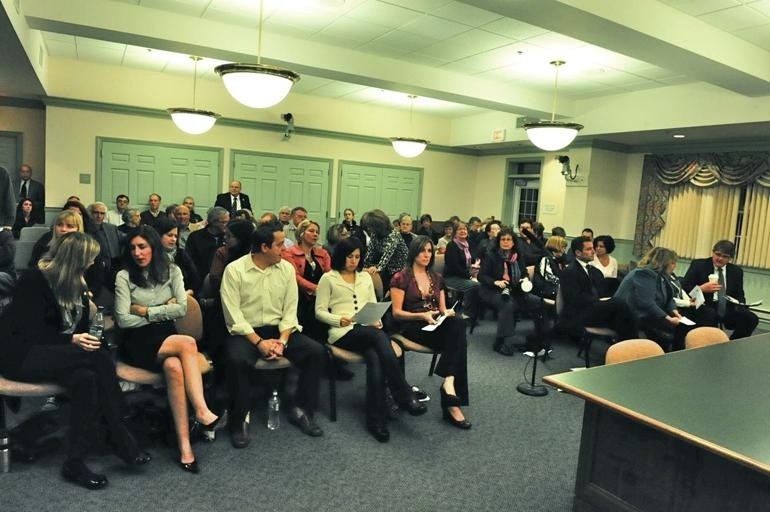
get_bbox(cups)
[707,273,719,284]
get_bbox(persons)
[0,165,471,491]
[443,217,759,353]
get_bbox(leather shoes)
[443,406,472,428]
[110,435,152,465]
[398,396,427,415]
[440,383,464,402]
[323,364,354,379]
[230,421,250,447]
[367,415,389,442]
[61,463,107,490]
[288,407,323,436]
[493,343,512,356]
[572,336,590,349]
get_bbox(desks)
[541,333,770,512]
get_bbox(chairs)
[12,241,37,273]
[20,227,49,241]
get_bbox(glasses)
[224,232,236,239]
[94,212,104,214]
[345,213,351,216]
[501,238,512,241]
[402,223,410,226]
[714,252,729,259]
[119,200,127,203]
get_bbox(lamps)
[166,56,221,135]
[214,0,300,108]
[522,58,583,151]
[388,94,430,158]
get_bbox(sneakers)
[42,397,60,411]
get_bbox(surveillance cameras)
[556,154,579,177]
[279,111,296,141]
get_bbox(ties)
[586,264,598,298]
[21,182,26,198]
[718,267,726,317]
[232,198,237,216]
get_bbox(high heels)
[194,412,219,430]
[176,441,197,472]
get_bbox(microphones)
[520,226,558,262]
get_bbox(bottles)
[88,306,105,352]
[266,388,280,431]
[0,436,12,474]
